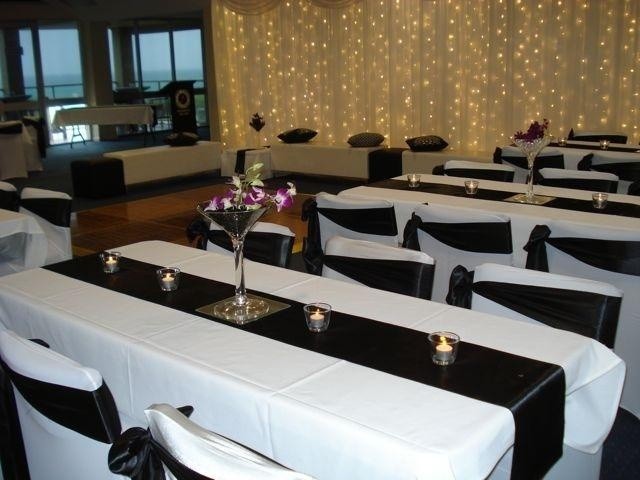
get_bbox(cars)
[558,137,567,147]
[600,140,610,150]
[100,251,121,274]
[303,302,332,332]
[592,193,608,209]
[464,181,479,195]
[156,267,181,292]
[428,331,460,367]
[407,176,421,187]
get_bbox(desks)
[54,104,159,147]
[2,209,47,277]
[335,172,639,271]
[3,239,626,479]
[2,114,46,183]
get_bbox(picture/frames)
[54,104,159,147]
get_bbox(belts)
[196,200,271,320]
[512,138,550,203]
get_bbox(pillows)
[408,135,448,154]
[275,127,318,146]
[169,131,201,147]
[348,131,385,149]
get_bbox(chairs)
[432,128,640,194]
[206,219,294,269]
[320,236,435,300]
[525,221,639,415]
[301,191,401,278]
[402,203,516,304]
[2,324,131,480]
[448,262,625,353]
[109,402,316,480]
[18,188,71,266]
[2,181,20,213]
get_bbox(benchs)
[265,141,406,183]
[79,139,223,199]
